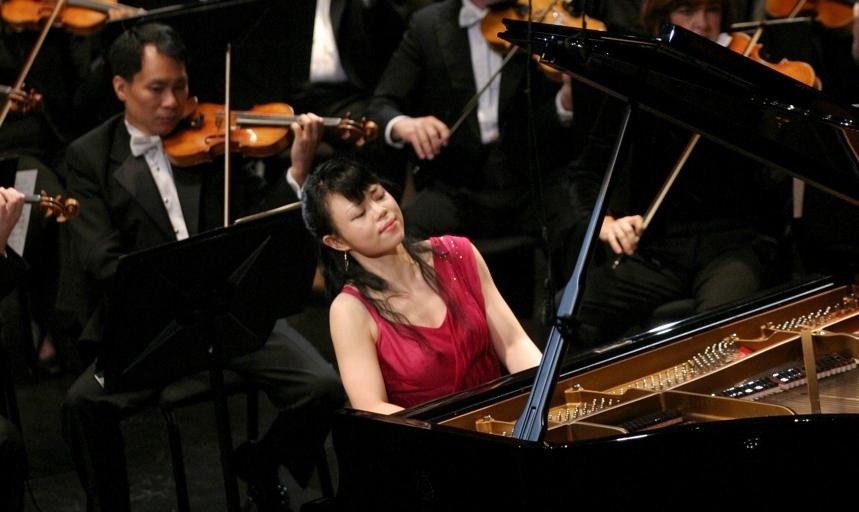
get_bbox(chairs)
[149,365,335,512]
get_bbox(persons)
[0,0,859,369]
[299,157,543,417]
[56,20,346,510]
[0,183,28,512]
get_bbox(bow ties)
[129,131,162,160]
[458,2,492,28]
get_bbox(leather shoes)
[233,436,293,511]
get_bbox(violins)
[481,0,606,75]
[164,104,376,167]
[0,0,246,36]
[719,31,822,90]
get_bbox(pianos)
[332,18,858,511]
